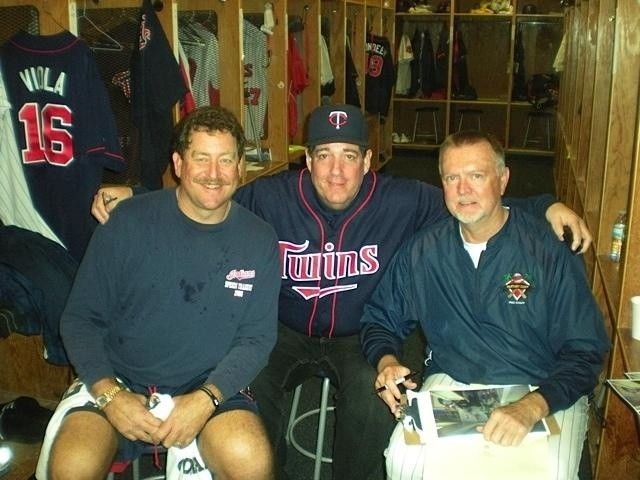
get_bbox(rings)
[175,440,181,446]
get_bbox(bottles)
[610,210,626,264]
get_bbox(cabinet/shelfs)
[156,1,397,196]
[397,2,568,155]
[553,1,640,480]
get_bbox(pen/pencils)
[372,368,423,395]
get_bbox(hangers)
[9,7,142,52]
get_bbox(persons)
[45,107,282,479]
[92,104,591,480]
[360,132,609,479]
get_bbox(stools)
[104,441,169,479]
[285,362,342,479]
[411,107,440,143]
[455,108,483,132]
[521,111,556,147]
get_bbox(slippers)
[0,396,54,444]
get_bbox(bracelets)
[199,387,219,409]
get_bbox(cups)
[631,296,640,341]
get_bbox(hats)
[303,105,369,146]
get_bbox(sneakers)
[392,132,411,144]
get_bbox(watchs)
[95,383,125,410]
